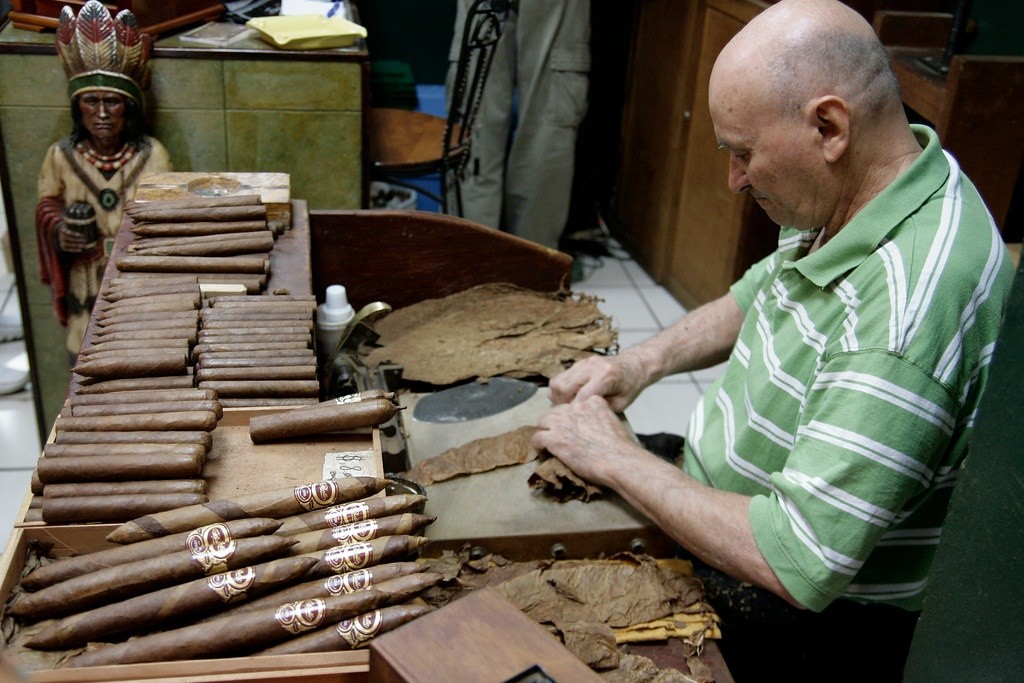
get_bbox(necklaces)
[78,138,136,170]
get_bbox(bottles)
[316,285,354,359]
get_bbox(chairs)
[364,0,512,218]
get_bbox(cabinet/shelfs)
[601,1,781,312]
[0,16,371,454]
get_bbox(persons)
[449,0,589,248]
[534,0,1017,683]
[35,0,173,366]
